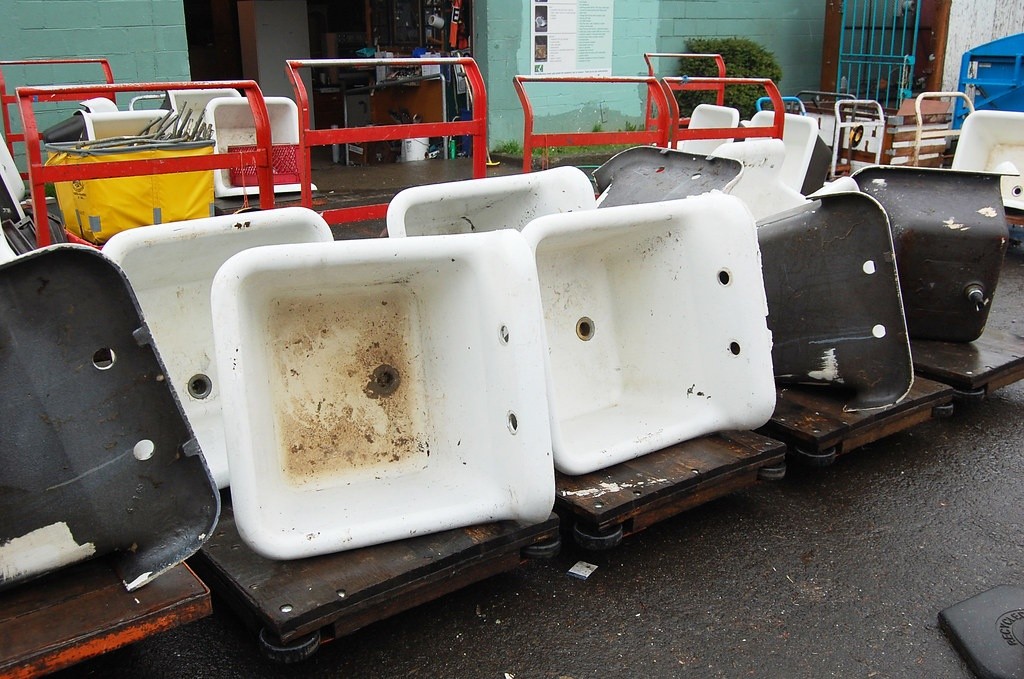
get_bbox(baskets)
[228,144,301,186]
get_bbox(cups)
[450,140,456,159]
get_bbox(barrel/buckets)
[401,138,429,161]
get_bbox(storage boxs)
[44,132,216,244]
[228,144,301,187]
